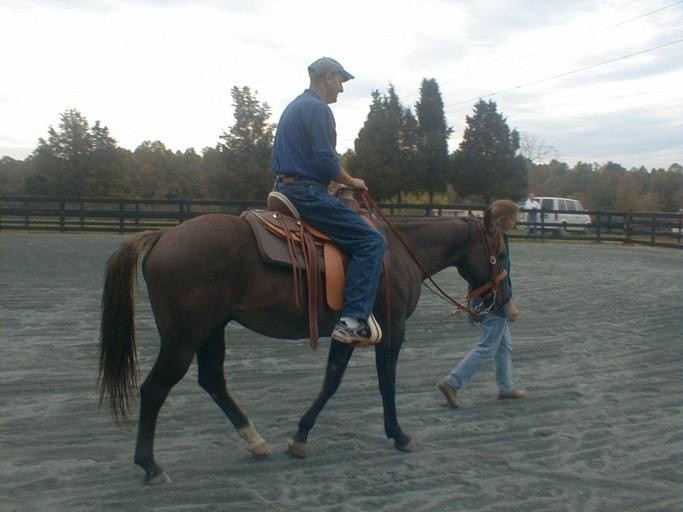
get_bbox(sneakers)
[332,317,371,343]
[438,380,462,406]
[497,390,527,400]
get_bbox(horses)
[95,206,513,487]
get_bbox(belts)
[276,173,309,184]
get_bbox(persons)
[438,199,526,409]
[525,192,541,235]
[273,56,389,343]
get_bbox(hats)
[307,55,353,81]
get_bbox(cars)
[523,197,590,235]
